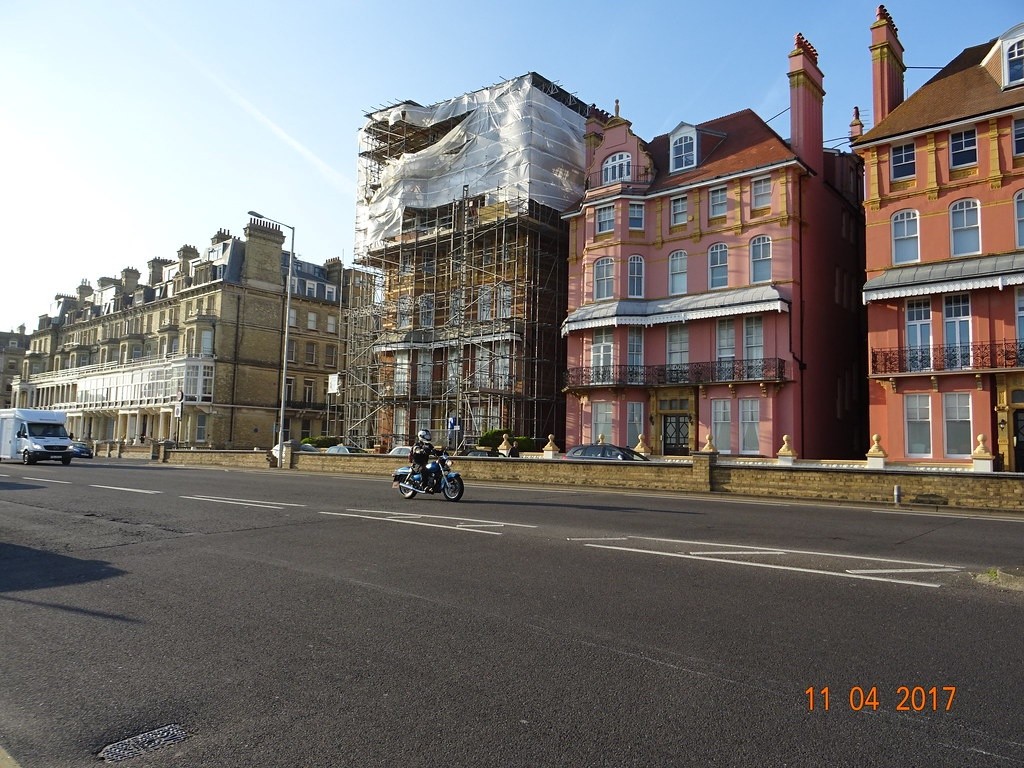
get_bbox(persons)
[411,429,449,494]
[508,441,519,457]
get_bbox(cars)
[466,450,507,458]
[326,446,368,454]
[388,446,412,455]
[562,443,651,462]
[73,442,94,459]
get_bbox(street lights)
[246,209,296,467]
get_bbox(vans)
[0,408,75,466]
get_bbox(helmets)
[418,430,432,444]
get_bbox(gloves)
[444,452,449,456]
[424,448,431,454]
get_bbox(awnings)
[560,284,790,338]
[863,251,1024,305]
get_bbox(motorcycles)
[391,446,464,501]
